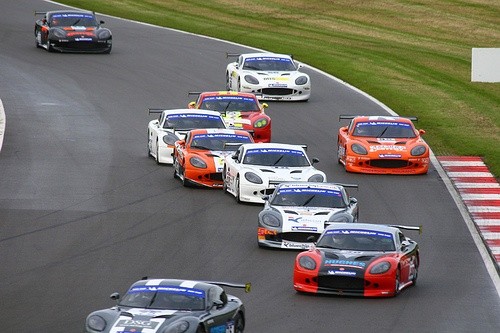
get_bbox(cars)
[337,115,430,175]
[34,9,112,54]
[188,91,271,143]
[293,221,422,297]
[257,180,359,250]
[225,53,311,102]
[173,128,263,189]
[85,276,251,332]
[147,108,228,165]
[222,143,327,204]
